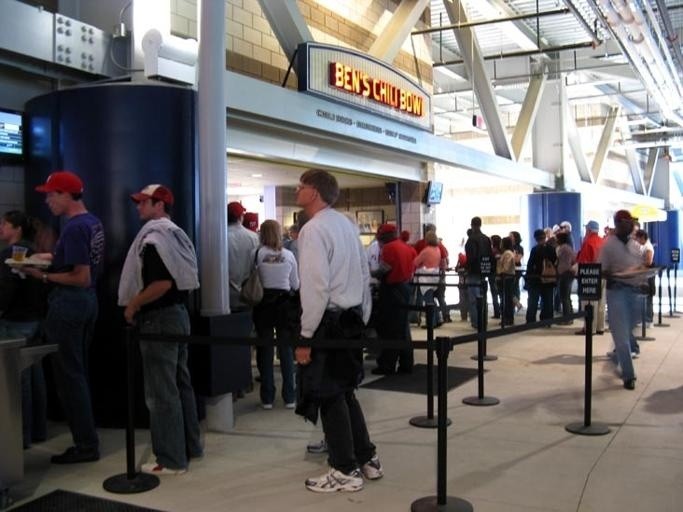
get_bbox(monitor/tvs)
[426,181,443,205]
[0,107,26,164]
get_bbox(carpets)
[362,363,490,395]
[5,488,163,512]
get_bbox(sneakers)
[49,445,102,464]
[361,453,385,481]
[303,468,367,495]
[573,329,597,336]
[304,438,328,453]
[140,462,189,478]
[597,330,604,335]
[623,379,636,390]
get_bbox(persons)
[227,202,260,398]
[19,170,104,464]
[362,226,383,360]
[455,216,610,335]
[294,168,384,493]
[597,210,643,390]
[118,183,204,476]
[636,230,655,329]
[375,225,418,377]
[414,223,453,323]
[0,210,47,449]
[288,226,299,254]
[413,230,444,330]
[249,220,300,410]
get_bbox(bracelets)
[41,271,47,283]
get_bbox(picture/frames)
[355,209,384,235]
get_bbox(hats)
[35,170,85,197]
[243,212,259,231]
[227,202,247,220]
[373,223,399,240]
[586,221,601,233]
[130,183,174,208]
[614,210,636,225]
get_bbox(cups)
[12,246,29,261]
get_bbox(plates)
[5,261,51,265]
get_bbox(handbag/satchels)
[239,266,265,306]
